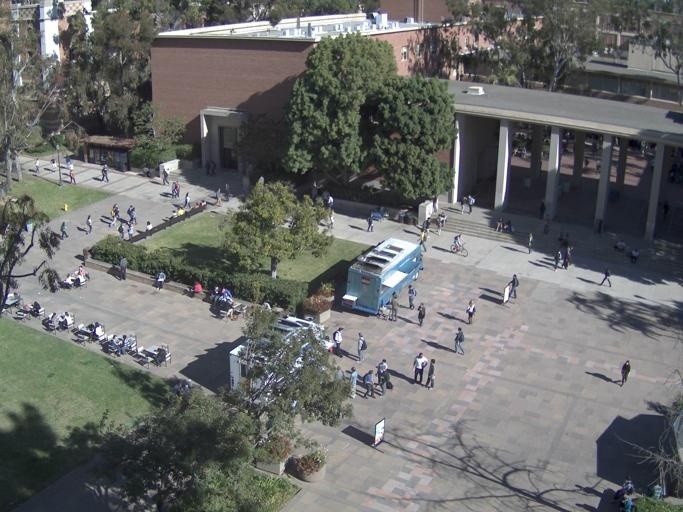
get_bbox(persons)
[629,247,640,262]
[454,327,464,354]
[357,332,367,362]
[416,227,428,252]
[221,187,230,202]
[204,159,210,176]
[108,203,136,239]
[77,266,85,276]
[62,311,72,330]
[64,272,75,286]
[153,270,167,290]
[614,238,626,252]
[153,347,166,367]
[120,334,129,353]
[50,312,62,331]
[366,211,375,232]
[85,214,92,234]
[407,284,416,309]
[599,264,612,286]
[553,232,575,271]
[390,295,398,321]
[539,199,545,220]
[59,220,69,239]
[31,301,41,317]
[201,199,206,206]
[411,351,428,386]
[49,156,56,175]
[333,358,393,398]
[214,187,223,207]
[209,159,216,176]
[525,231,535,255]
[507,273,519,299]
[435,213,447,235]
[465,299,476,324]
[64,156,72,170]
[118,255,127,281]
[171,187,177,199]
[454,232,464,250]
[76,272,85,285]
[459,196,467,215]
[423,358,436,390]
[90,322,102,343]
[34,156,40,173]
[145,220,152,231]
[161,168,169,185]
[198,202,202,207]
[207,284,233,317]
[171,210,176,218]
[331,327,343,352]
[495,216,504,232]
[325,194,334,208]
[6,291,20,309]
[69,169,77,185]
[543,212,550,232]
[422,217,431,238]
[183,192,191,208]
[174,180,180,198]
[194,202,199,208]
[100,163,109,183]
[613,473,663,512]
[187,279,202,299]
[417,302,426,327]
[321,335,333,354]
[111,334,120,356]
[620,360,630,386]
[467,195,475,214]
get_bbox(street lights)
[50,143,64,186]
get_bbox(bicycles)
[449,243,468,257]
